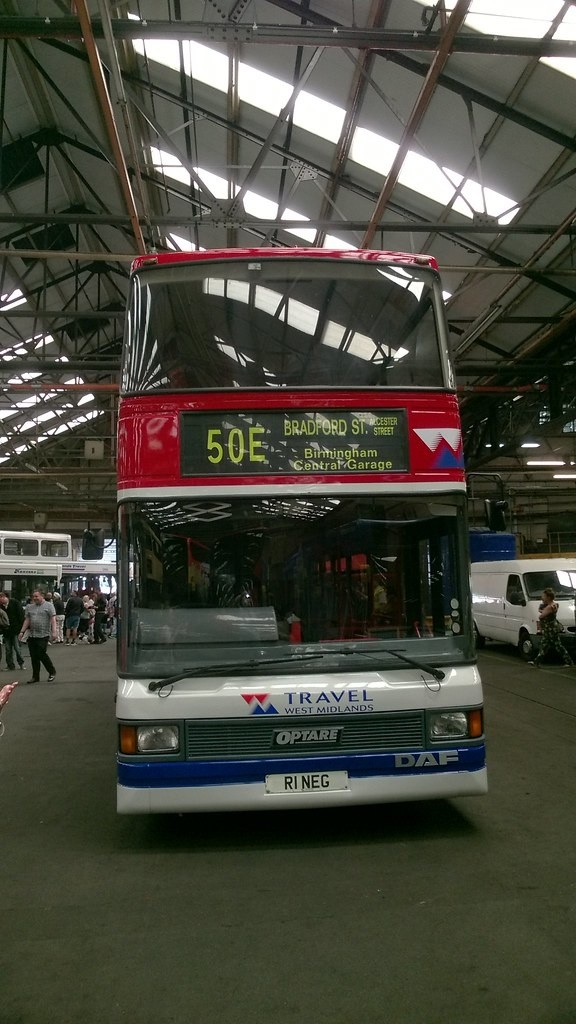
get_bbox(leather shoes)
[27,678,40,683]
[48,675,55,681]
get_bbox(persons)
[14,586,118,646]
[0,682,19,714]
[0,592,27,670]
[527,587,574,669]
[17,589,57,684]
[0,608,10,664]
[353,561,394,635]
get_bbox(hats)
[54,592,61,598]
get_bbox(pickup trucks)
[0,529,135,589]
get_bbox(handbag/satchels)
[554,619,566,634]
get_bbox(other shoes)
[65,641,70,645]
[91,640,99,644]
[528,661,540,668]
[71,641,77,645]
[2,667,15,671]
[99,638,107,644]
[19,665,26,669]
[561,663,575,668]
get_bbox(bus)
[113,247,508,815]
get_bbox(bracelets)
[19,631,25,635]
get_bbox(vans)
[469,556,575,659]
